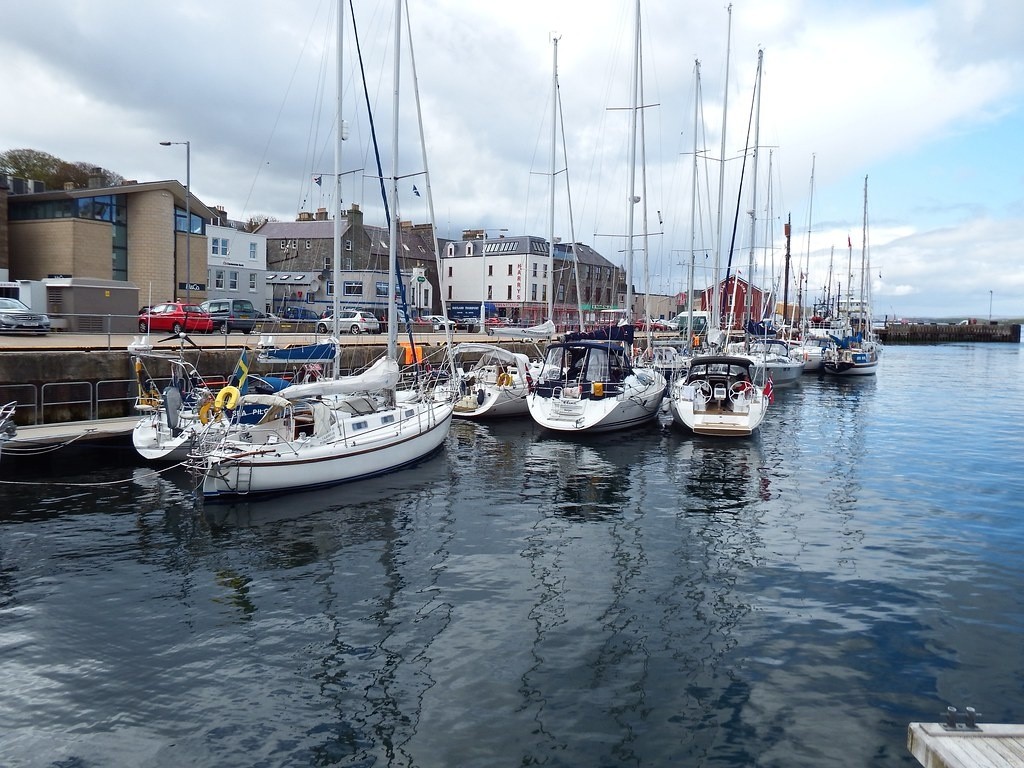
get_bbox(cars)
[485,318,504,328]
[421,316,446,330]
[316,311,379,335]
[633,318,667,332]
[319,309,334,318]
[436,316,455,330]
[284,306,318,319]
[0,298,51,335]
[139,303,213,335]
[653,319,677,331]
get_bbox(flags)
[235,350,249,396]
[525,366,534,395]
[763,375,775,405]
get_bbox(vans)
[667,311,712,331]
[194,299,256,334]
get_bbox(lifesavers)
[214,386,240,411]
[496,372,510,387]
[199,400,224,425]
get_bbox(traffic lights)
[417,276,425,282]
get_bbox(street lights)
[159,141,190,304]
[989,291,992,325]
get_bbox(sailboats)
[133,1,468,496]
[526,0,667,433]
[631,2,883,388]
[400,1,568,416]
[670,48,774,436]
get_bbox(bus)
[520,304,634,332]
